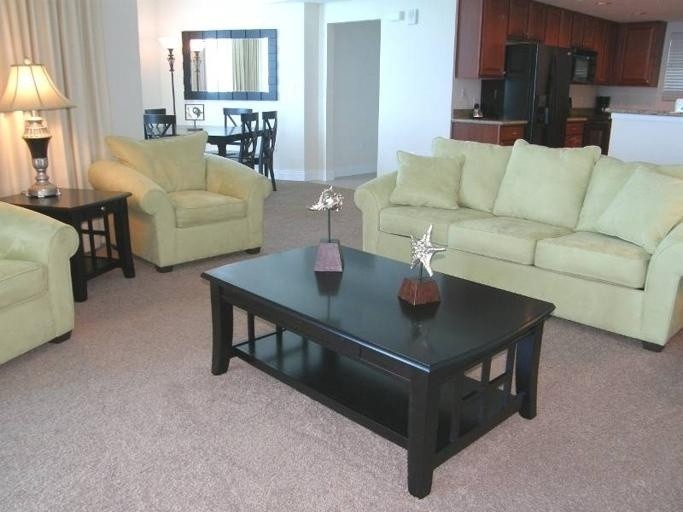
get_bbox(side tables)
[0,188,135,302]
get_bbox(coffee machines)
[595,97,611,120]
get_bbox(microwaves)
[568,48,597,85]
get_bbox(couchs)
[353,170,683,352]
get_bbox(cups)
[674,97,683,113]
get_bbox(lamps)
[190,42,204,91]
[159,38,180,135]
[0,56,78,198]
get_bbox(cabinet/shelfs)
[453,0,667,88]
[498,126,526,145]
[565,121,585,147]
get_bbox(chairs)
[88,154,273,272]
[0,201,80,365]
[143,108,277,191]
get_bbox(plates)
[668,109,683,116]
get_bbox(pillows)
[431,137,514,213]
[390,150,466,210]
[574,154,683,233]
[595,165,683,254]
[107,132,209,193]
[493,139,601,229]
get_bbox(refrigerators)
[494,41,574,149]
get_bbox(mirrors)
[181,29,277,101]
